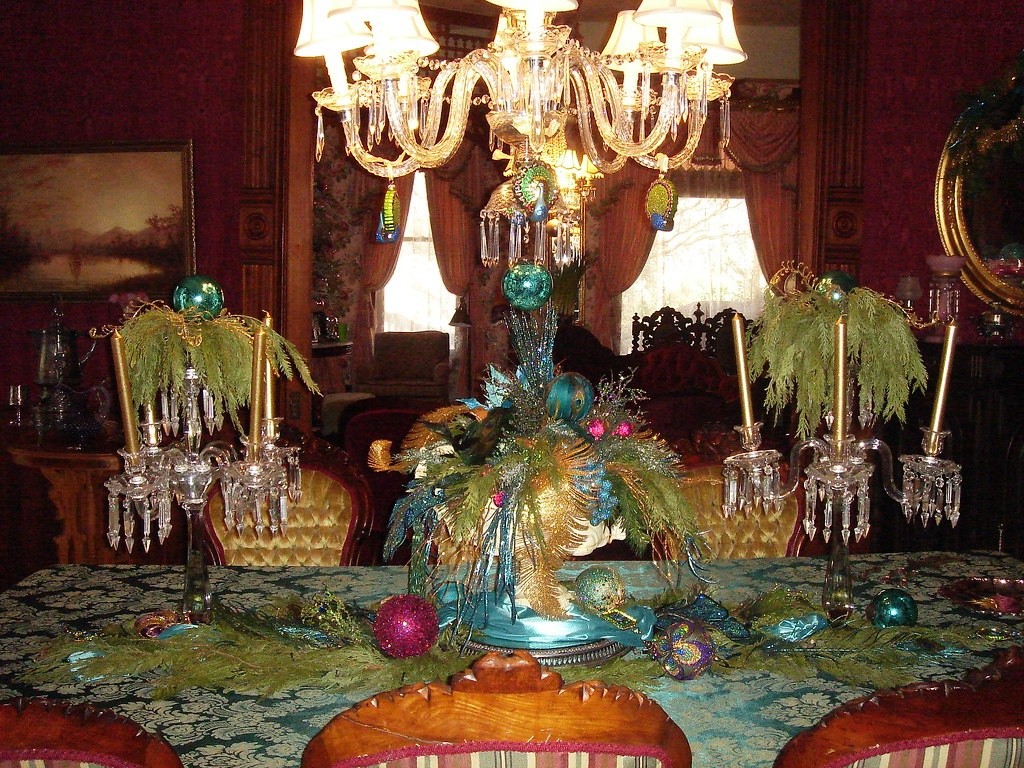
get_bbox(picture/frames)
[0,138,197,305]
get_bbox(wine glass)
[8,384,28,427]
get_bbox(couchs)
[358,330,449,409]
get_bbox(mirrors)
[933,74,1024,320]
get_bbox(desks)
[0,545,1024,768]
[0,410,247,589]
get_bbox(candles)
[732,311,757,443]
[110,327,140,464]
[834,314,846,458]
[142,397,159,445]
[926,314,958,451]
[261,309,278,439]
[247,326,266,458]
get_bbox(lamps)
[293,0,749,267]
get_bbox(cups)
[338,323,348,343]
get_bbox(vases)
[412,447,637,669]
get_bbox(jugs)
[25,307,98,413]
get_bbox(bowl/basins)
[938,576,1024,622]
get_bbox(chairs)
[0,696,184,768]
[300,647,693,768]
[629,303,763,426]
[202,432,378,567]
[773,646,1024,768]
[644,428,808,563]
